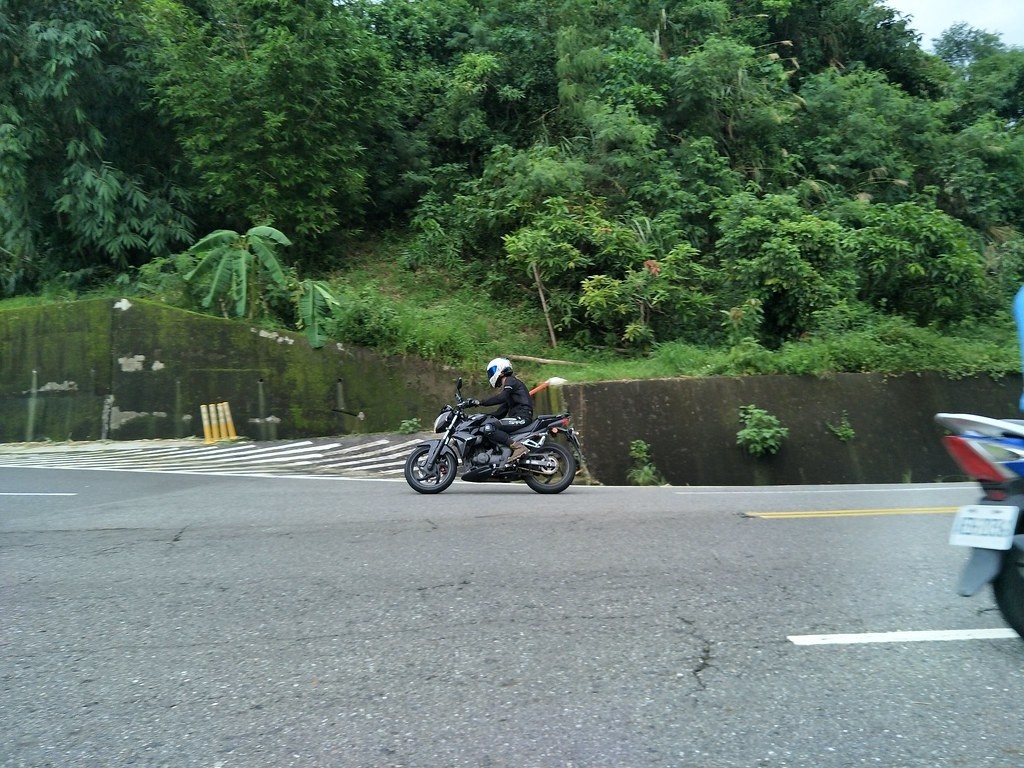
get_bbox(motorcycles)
[403,375,582,494]
[933,411,1024,636]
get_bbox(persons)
[470,357,533,463]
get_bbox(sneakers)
[508,440,531,462]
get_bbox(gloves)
[471,400,480,407]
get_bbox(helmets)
[486,357,514,389]
